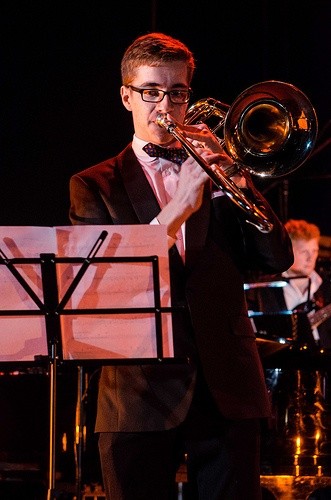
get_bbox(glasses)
[126,84,192,104]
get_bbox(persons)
[258,220,331,405]
[70,32,295,500]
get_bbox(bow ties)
[142,142,189,165]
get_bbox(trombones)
[152,79,320,239]
